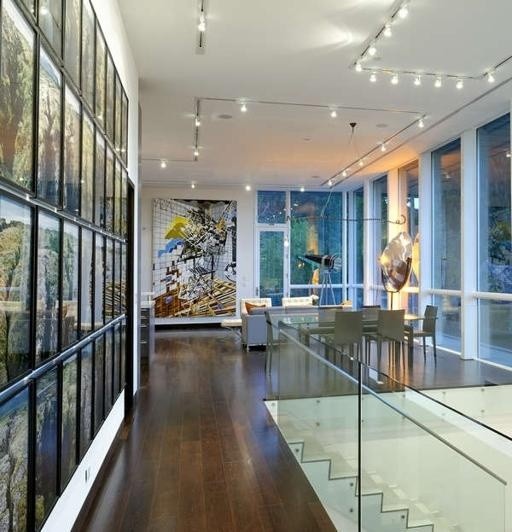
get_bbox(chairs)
[413,306,438,364]
[316,307,342,325]
[324,312,365,381]
[361,305,381,321]
[370,310,405,380]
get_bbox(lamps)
[197,0,214,48]
[159,94,428,192]
[351,1,496,88]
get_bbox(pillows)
[245,303,265,313]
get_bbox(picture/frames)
[1,381,35,531]
[34,0,131,530]
[1,189,33,401]
[1,0,39,199]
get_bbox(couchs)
[227,294,320,353]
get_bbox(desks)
[239,309,318,351]
[303,313,440,379]
